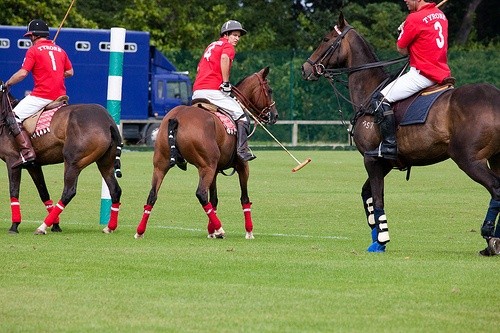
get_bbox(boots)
[364,96,398,161]
[9,123,36,168]
[236,121,256,162]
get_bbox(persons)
[364,0,450,160]
[5,19,73,169]
[192,20,256,162]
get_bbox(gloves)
[219,81,232,96]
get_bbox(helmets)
[23,19,50,37]
[220,20,248,37]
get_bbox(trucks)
[1,26,195,147]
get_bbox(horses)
[1,80,123,236]
[134,65,279,240]
[299,11,499,257]
